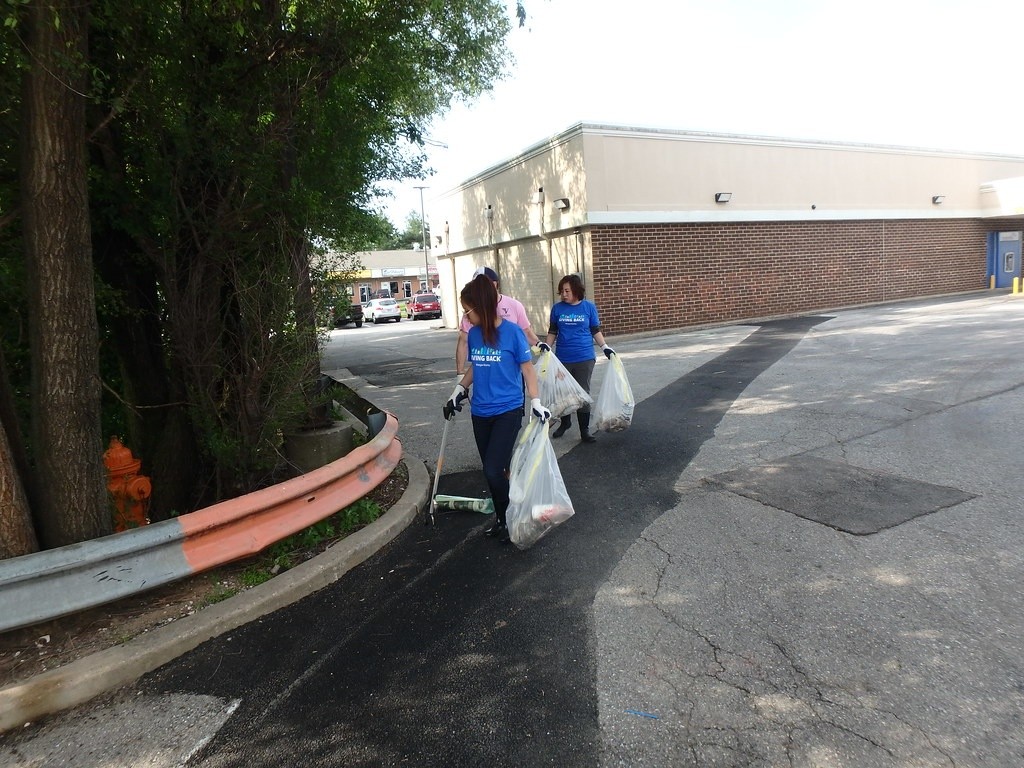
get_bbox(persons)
[446,274,552,543]
[457,265,551,383]
[545,273,616,442]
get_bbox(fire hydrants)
[100,434,153,533]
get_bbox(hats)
[473,266,498,281]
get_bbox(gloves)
[600,343,616,360]
[531,399,551,424]
[537,341,550,353]
[457,374,465,384]
[443,385,469,421]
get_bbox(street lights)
[413,186,431,295]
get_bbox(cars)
[362,298,402,324]
[404,293,441,321]
[416,284,441,303]
[371,288,390,299]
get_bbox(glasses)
[462,306,477,317]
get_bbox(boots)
[483,500,502,536]
[577,412,596,442]
[500,501,510,542]
[553,414,572,438]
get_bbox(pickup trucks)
[318,297,364,330]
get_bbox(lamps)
[554,199,569,209]
[933,195,946,203]
[715,193,732,202]
